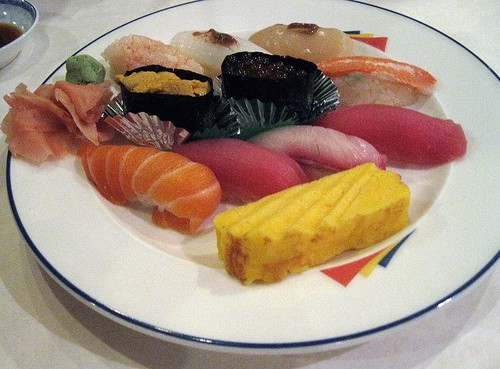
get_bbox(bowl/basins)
[0,0,39,70]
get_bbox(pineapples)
[212,163,410,285]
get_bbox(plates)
[6,1,500,355]
